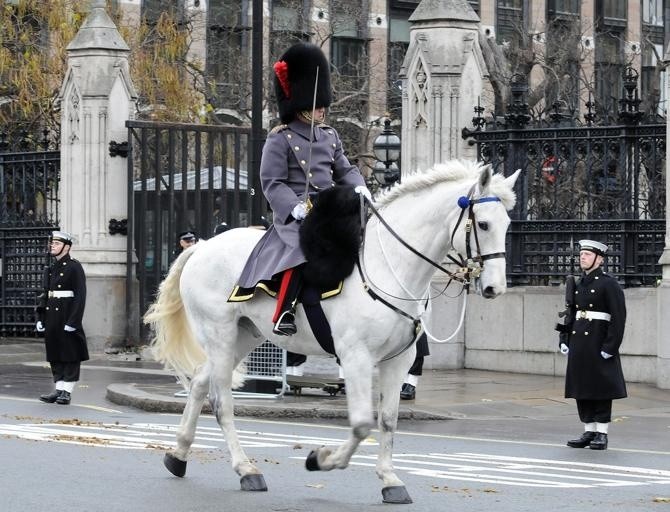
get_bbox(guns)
[555,242,575,347]
[35,235,52,328]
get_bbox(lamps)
[372,115,401,188]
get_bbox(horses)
[142,159,523,506]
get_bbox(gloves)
[291,201,309,220]
[600,351,614,360]
[558,341,570,354]
[64,325,75,332]
[355,184,371,201]
[36,320,44,333]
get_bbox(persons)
[557,239,629,450]
[177,228,197,251]
[34,230,90,404]
[255,43,375,335]
[212,222,232,236]
[395,294,433,401]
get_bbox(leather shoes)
[39,390,61,403]
[56,389,71,404]
[590,430,609,449]
[272,312,298,336]
[399,383,416,400]
[566,431,595,449]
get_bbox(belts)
[46,290,74,298]
[575,310,611,323]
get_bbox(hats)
[50,230,73,246]
[577,238,609,257]
[178,230,197,242]
[275,43,332,122]
